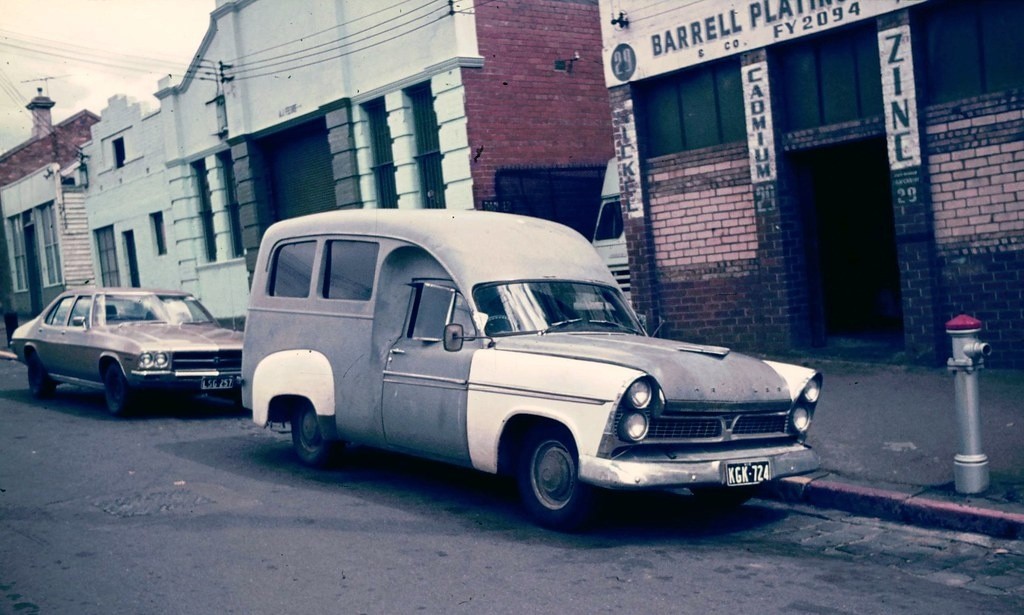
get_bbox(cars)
[10,288,245,414]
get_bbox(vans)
[238,207,826,529]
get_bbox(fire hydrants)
[945,314,993,494]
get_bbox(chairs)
[106,304,118,323]
[145,297,169,320]
